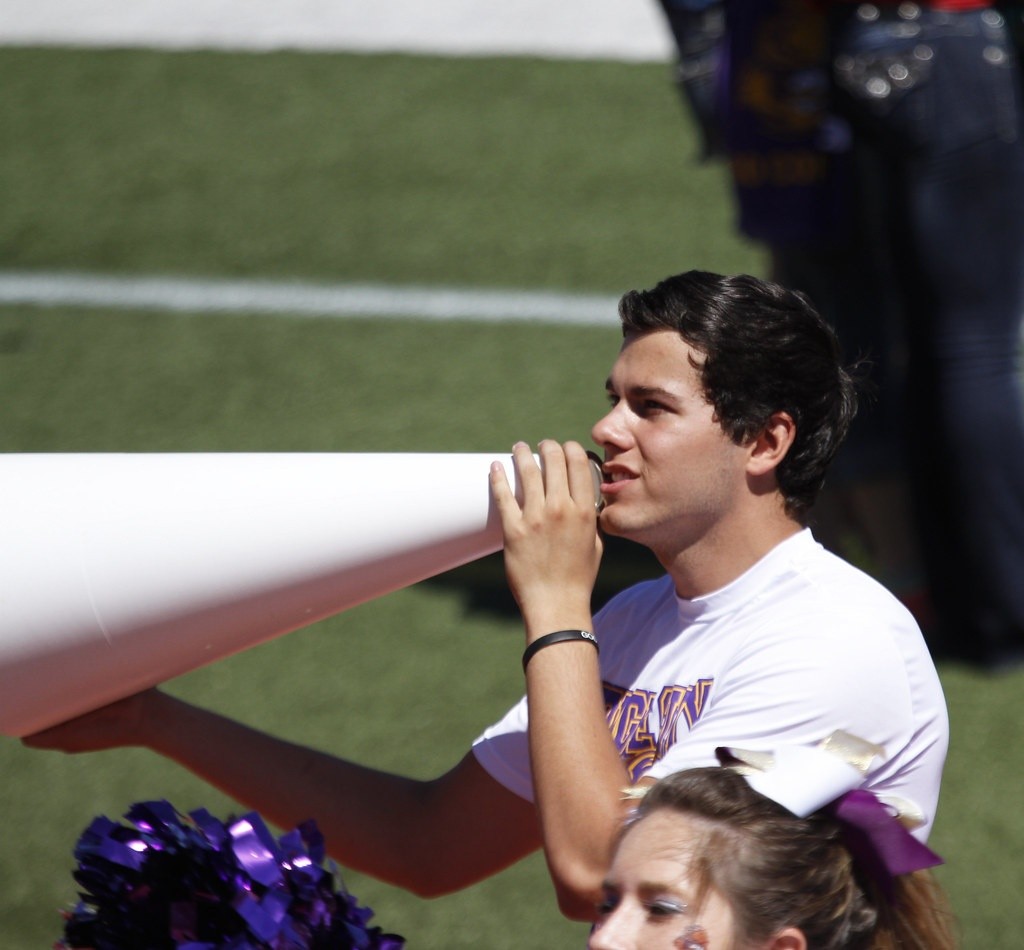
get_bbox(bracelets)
[521,630,600,672]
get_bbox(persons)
[585,730,967,950]
[18,269,951,925]
